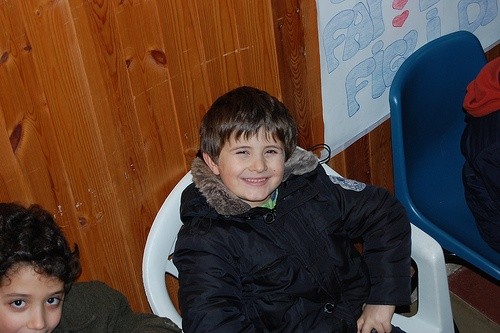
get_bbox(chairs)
[141,141,455,333]
[389,30,500,287]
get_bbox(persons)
[461,56,500,259]
[173,86,411,333]
[0,202,184,333]
